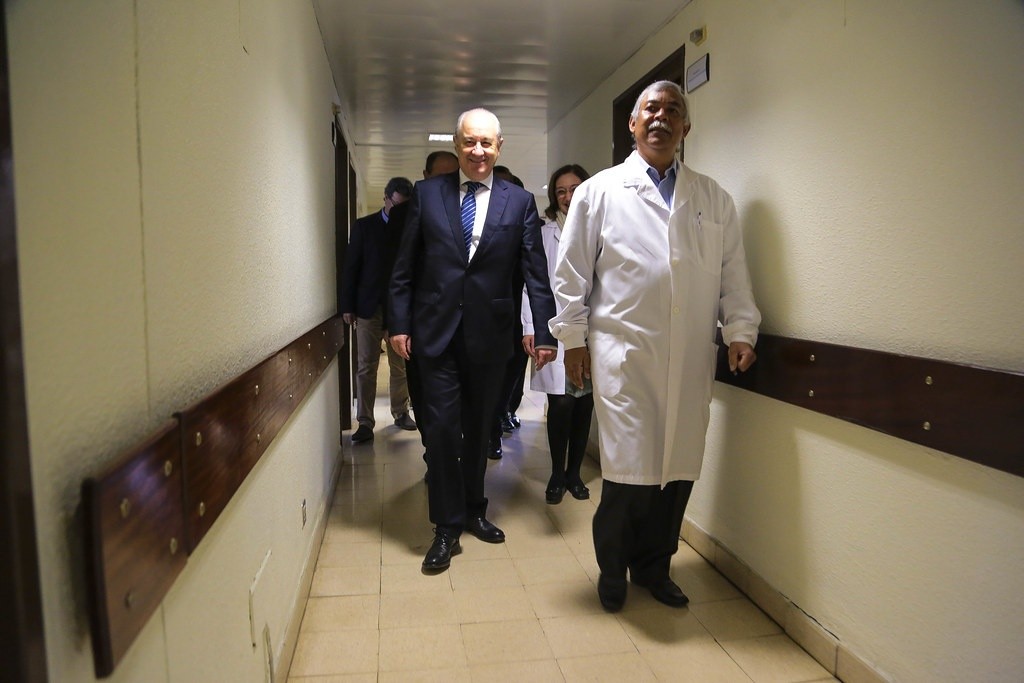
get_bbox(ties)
[460,181,485,263]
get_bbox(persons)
[337,150,594,505]
[547,80,762,613]
[385,107,558,569]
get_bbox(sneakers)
[394,414,417,430]
[352,425,374,441]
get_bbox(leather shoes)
[598,573,628,612]
[468,518,505,542]
[502,415,521,430]
[631,574,690,607]
[488,444,502,459]
[423,528,460,569]
[545,472,566,504]
[565,470,589,500]
